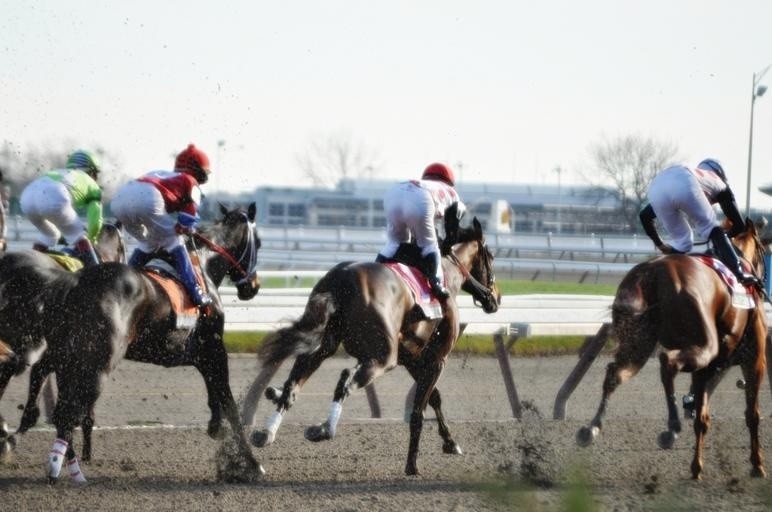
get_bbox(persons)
[375,163,466,298]
[639,159,761,287]
[111,143,213,308]
[20,148,103,266]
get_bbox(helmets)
[697,158,727,181]
[173,144,210,173]
[422,163,454,186]
[65,150,100,173]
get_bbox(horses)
[0,219,128,455]
[574,215,768,479]
[242,216,502,476]
[17,202,266,486]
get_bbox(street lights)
[745,63,771,212]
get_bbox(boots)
[76,237,99,268]
[372,252,390,264]
[171,245,211,308]
[715,233,753,284]
[424,251,450,298]
[123,250,148,268]
